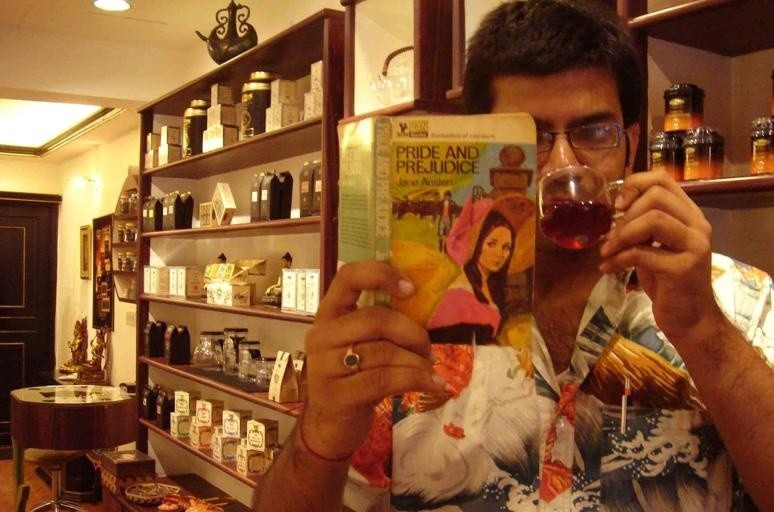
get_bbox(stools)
[23,446,87,512]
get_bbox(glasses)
[536,123,628,154]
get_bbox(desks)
[9,384,133,503]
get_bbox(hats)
[444,192,536,274]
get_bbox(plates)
[122,482,182,505]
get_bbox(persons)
[252,0,774,512]
[427,193,536,346]
[432,191,460,253]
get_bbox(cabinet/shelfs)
[109,164,138,304]
[134,6,343,492]
[615,0,774,211]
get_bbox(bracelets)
[299,410,353,463]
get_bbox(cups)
[536,162,628,249]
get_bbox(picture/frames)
[78,223,91,279]
[91,213,116,332]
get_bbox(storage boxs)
[99,451,156,497]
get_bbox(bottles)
[179,99,205,157]
[650,83,723,181]
[238,72,271,139]
[191,328,276,388]
[750,116,773,174]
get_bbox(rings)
[343,344,361,373]
